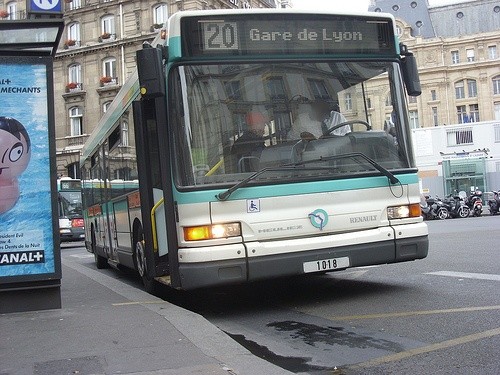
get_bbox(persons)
[286,89,352,143]
[230,105,274,172]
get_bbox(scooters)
[485,189,500,215]
[420,187,484,220]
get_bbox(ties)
[321,122,328,135]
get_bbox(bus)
[56,175,85,243]
[78,7,431,294]
